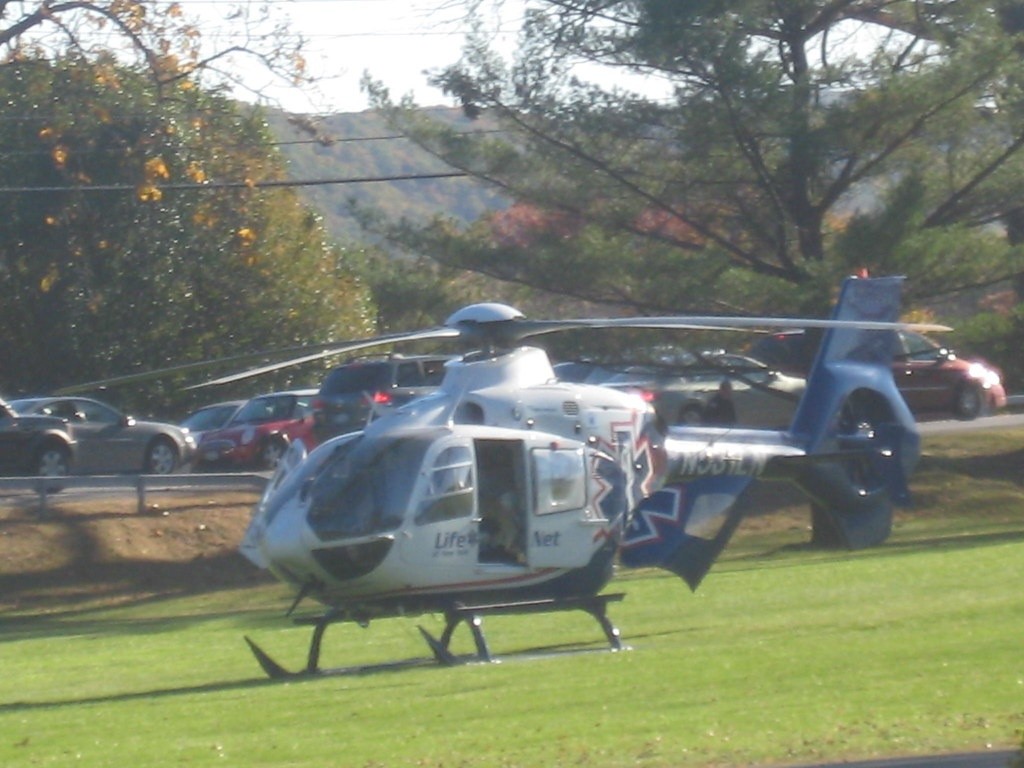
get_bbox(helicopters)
[50,274,956,680]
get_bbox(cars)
[0,398,80,495]
[9,397,198,476]
[171,350,462,474]
[549,323,1008,432]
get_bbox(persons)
[706,382,736,424]
